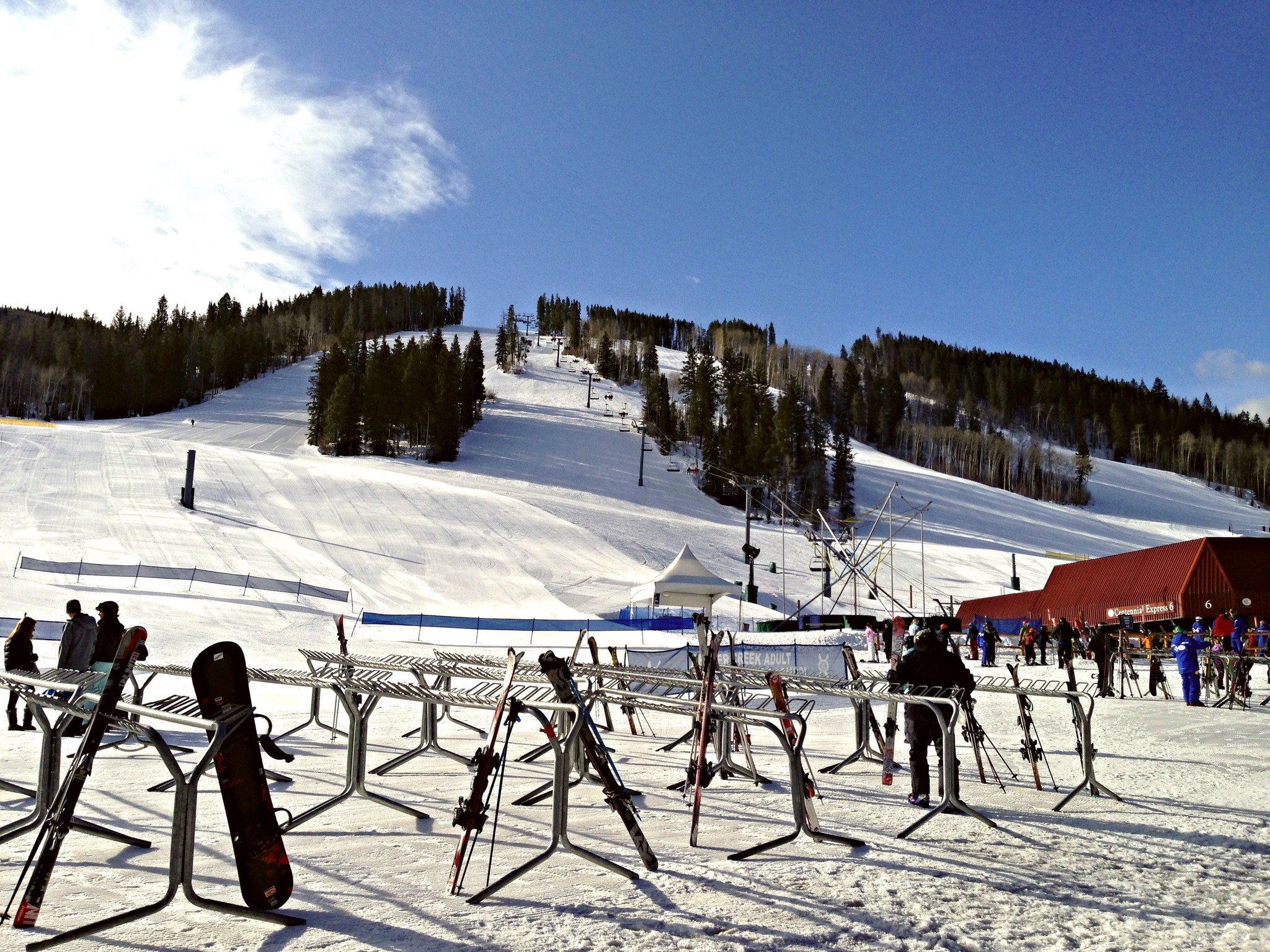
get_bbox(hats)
[95,601,118,616]
[66,599,81,613]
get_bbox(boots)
[23,708,36,730]
[6,708,24,731]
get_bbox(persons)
[54,599,98,738]
[191,419,195,427]
[79,601,129,736]
[937,623,950,646]
[4,616,40,731]
[865,621,893,664]
[1192,616,1205,655]
[964,621,1002,667]
[908,619,920,637]
[1018,618,1074,670]
[886,626,978,815]
[1212,607,1268,658]
[1086,621,1115,696]
[1171,624,1213,707]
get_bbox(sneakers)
[1025,661,1048,666]
[981,662,997,667]
[940,798,966,814]
[1190,701,1204,706]
[908,793,928,808]
[1186,702,1190,705]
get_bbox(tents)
[630,543,743,632]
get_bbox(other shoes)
[970,658,978,660]
[61,731,74,737]
[1108,692,1114,697]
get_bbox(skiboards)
[948,636,986,784]
[1156,659,1169,700]
[11,625,147,927]
[1040,663,1054,666]
[1205,656,1221,698]
[690,630,724,848]
[979,666,1001,667]
[859,659,886,665]
[882,616,904,785]
[538,649,659,872]
[965,659,982,660]
[1021,664,1041,666]
[1122,646,1144,698]
[881,660,893,663]
[1062,656,1100,797]
[1006,662,1043,791]
[765,670,823,843]
[1228,658,1242,710]
[444,647,525,896]
[841,647,886,756]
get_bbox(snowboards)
[1149,655,1157,697]
[190,642,294,909]
[1073,636,1087,660]
[1075,618,1092,660]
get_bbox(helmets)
[968,622,974,626]
[1175,625,1185,633]
[941,624,947,629]
[1098,622,1109,630]
[1041,626,1047,631]
[1196,617,1202,621]
[1060,618,1066,622]
[1023,620,1029,625]
[1029,628,1037,635]
